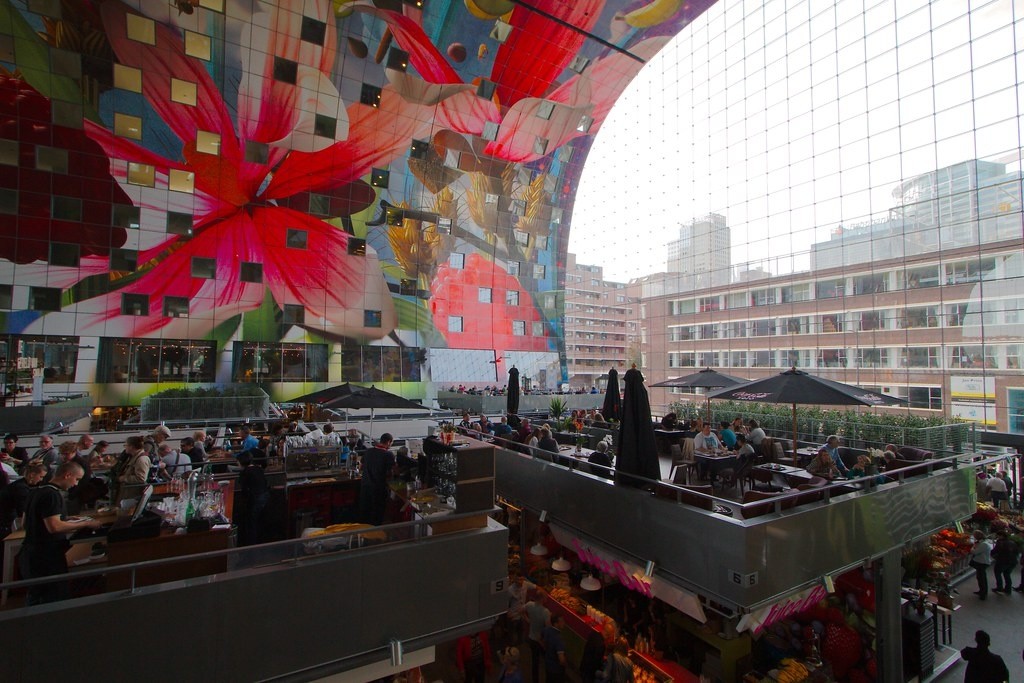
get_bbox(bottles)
[634,633,650,655]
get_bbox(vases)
[908,556,971,590]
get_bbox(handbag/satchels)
[968,559,977,569]
[119,452,150,504]
[79,466,108,504]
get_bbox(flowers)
[903,501,1023,580]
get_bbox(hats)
[149,425,171,437]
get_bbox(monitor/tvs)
[130,485,154,523]
[395,451,419,483]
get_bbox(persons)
[449,384,606,397]
[970,528,1024,600]
[570,408,621,431]
[751,634,797,666]
[662,413,767,486]
[0,426,278,586]
[960,629,1009,683]
[975,470,1013,512]
[805,434,907,486]
[456,543,654,683]
[588,440,612,479]
[321,423,420,545]
[461,413,560,462]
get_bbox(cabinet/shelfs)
[425,432,495,514]
[385,479,488,541]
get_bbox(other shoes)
[992,588,1004,593]
[974,591,987,600]
[1004,589,1011,596]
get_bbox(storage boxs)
[702,650,721,683]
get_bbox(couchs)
[838,447,870,471]
[656,481,733,517]
[795,476,826,506]
[741,483,800,519]
[884,447,933,480]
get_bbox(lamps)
[530,522,601,591]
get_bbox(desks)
[902,587,962,648]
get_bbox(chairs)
[663,438,794,497]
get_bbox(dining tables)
[557,444,617,477]
[552,430,596,448]
[693,453,736,487]
[1,446,270,605]
[785,447,819,468]
[751,462,804,490]
[655,428,685,451]
[787,469,864,490]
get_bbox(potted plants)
[668,399,795,437]
[795,399,968,450]
[549,397,585,452]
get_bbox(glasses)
[58,452,72,457]
[123,444,130,448]
[39,440,49,443]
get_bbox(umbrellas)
[322,384,444,447]
[278,381,372,439]
[602,366,622,423]
[614,362,662,481]
[708,366,911,467]
[506,364,519,415]
[647,367,755,426]
